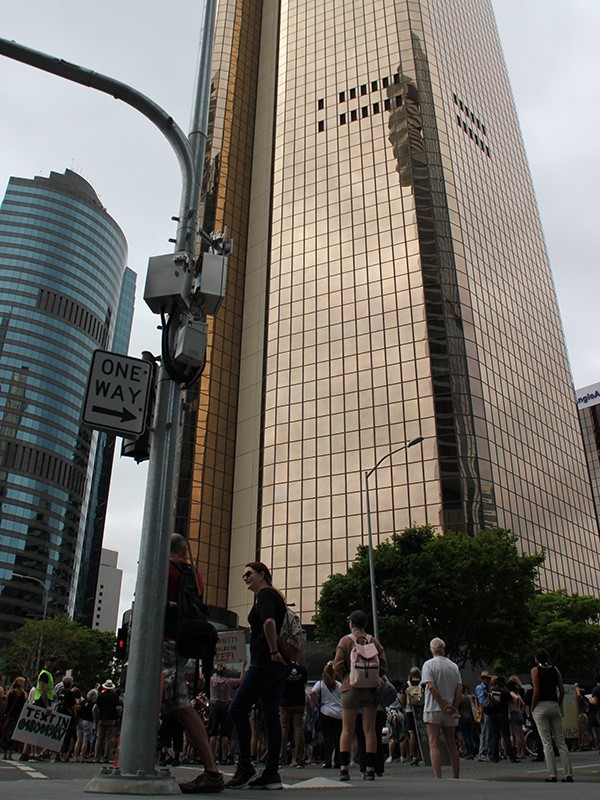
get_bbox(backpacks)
[256,585,307,661]
[405,679,424,708]
[169,559,219,660]
[346,633,379,688]
[380,681,398,708]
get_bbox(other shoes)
[365,767,376,781]
[18,754,31,762]
[545,776,557,782]
[178,770,224,794]
[386,756,395,764]
[225,761,258,789]
[401,756,409,763]
[339,769,350,781]
[247,769,283,790]
[561,775,574,783]
[410,757,420,767]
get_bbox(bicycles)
[524,729,540,757]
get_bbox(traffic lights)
[112,628,127,657]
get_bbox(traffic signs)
[82,349,153,437]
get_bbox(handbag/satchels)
[313,707,326,732]
[475,706,485,724]
[482,699,498,713]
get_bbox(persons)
[277,649,365,768]
[457,662,545,763]
[332,611,388,781]
[0,655,126,764]
[157,535,226,793]
[153,657,265,766]
[228,561,297,791]
[422,638,460,779]
[530,648,575,784]
[550,676,600,757]
[372,666,424,777]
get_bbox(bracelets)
[269,649,279,656]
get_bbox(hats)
[347,610,368,623]
[480,671,492,678]
[102,679,116,689]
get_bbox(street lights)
[11,572,48,679]
[364,437,427,642]
